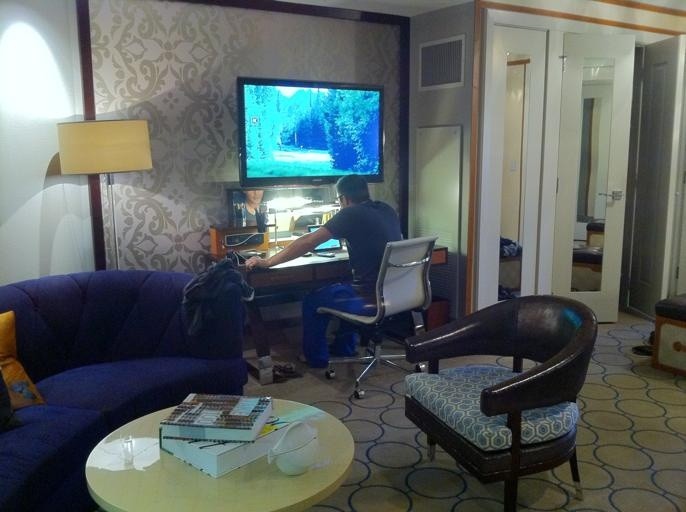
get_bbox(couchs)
[0,270,247,511]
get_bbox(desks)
[208,222,453,386]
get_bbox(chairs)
[402,294,600,511]
[315,235,441,399]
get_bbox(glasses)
[334,194,344,204]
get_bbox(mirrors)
[227,184,342,247]
[569,58,616,293]
[494,49,531,304]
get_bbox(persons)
[234,188,264,228]
[245,174,401,368]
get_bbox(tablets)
[307,224,342,252]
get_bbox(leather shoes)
[649,331,655,345]
[631,346,653,356]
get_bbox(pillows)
[0,369,20,429]
[0,311,46,409]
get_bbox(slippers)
[329,343,359,357]
[275,361,296,377]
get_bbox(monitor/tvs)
[237,75,384,187]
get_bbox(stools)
[499,237,522,293]
[651,292,685,378]
[571,247,605,292]
[585,217,604,248]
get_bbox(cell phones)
[317,251,335,257]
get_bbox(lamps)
[56,118,153,270]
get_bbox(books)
[162,393,271,443]
[159,419,289,479]
